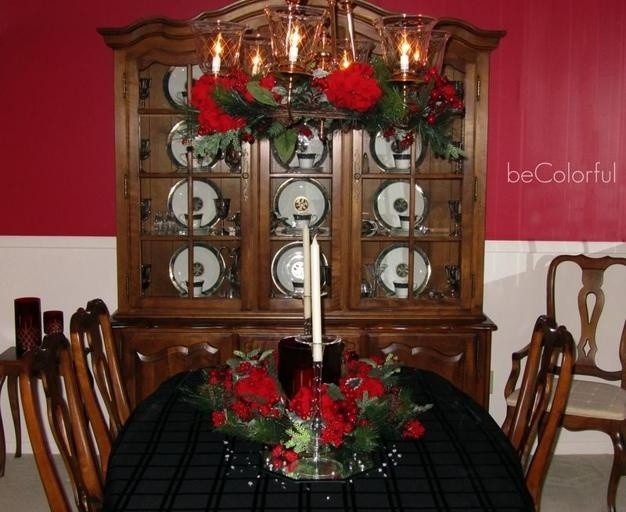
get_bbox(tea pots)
[215,243,242,298]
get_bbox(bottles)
[363,153,369,174]
[360,279,370,297]
[235,212,241,237]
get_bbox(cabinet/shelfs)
[112,316,497,418]
[95,0,509,314]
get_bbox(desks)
[0,344,92,476]
[98,362,534,511]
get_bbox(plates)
[372,124,433,298]
[163,64,204,108]
[274,176,330,230]
[271,243,330,296]
[273,121,328,170]
[166,180,223,229]
[168,240,226,294]
[165,120,221,168]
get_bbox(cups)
[291,280,305,295]
[42,311,64,335]
[292,213,319,229]
[296,152,316,169]
[13,297,43,360]
[179,278,205,296]
[154,211,178,235]
[362,220,378,238]
[184,213,202,230]
[141,263,152,293]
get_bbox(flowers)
[180,64,471,167]
[192,350,433,475]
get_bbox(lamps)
[178,0,470,167]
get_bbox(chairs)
[16,332,109,512]
[66,300,132,481]
[518,325,576,512]
[500,251,625,512]
[506,313,558,473]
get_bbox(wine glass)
[444,265,460,298]
[449,200,462,237]
[213,198,231,235]
[140,136,151,174]
[138,74,152,109]
[141,197,152,234]
[451,140,462,173]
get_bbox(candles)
[310,234,324,364]
[301,222,311,321]
